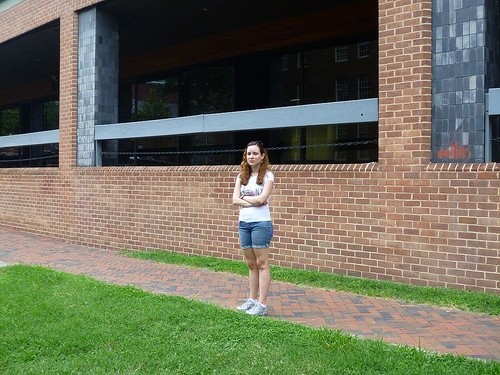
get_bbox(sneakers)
[245,302,268,316]
[236,298,257,311]
